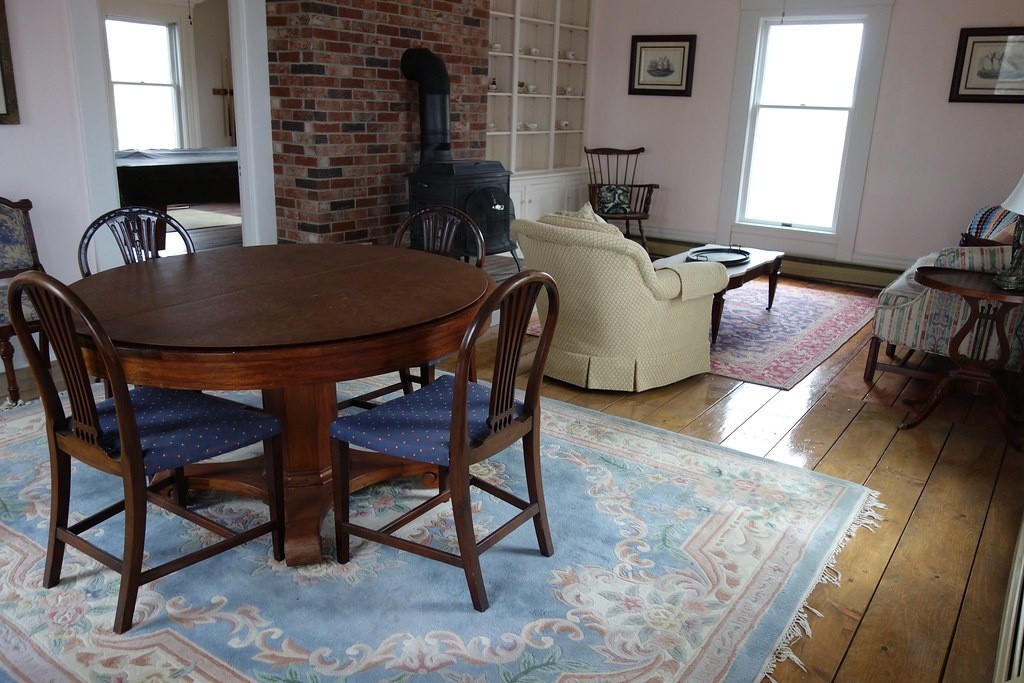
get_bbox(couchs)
[503,203,731,396]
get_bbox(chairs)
[0,198,53,402]
[80,207,210,402]
[583,146,660,253]
[10,269,286,635]
[863,204,1024,394]
[347,205,487,408]
[331,272,562,610]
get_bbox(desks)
[67,252,491,570]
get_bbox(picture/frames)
[0,0,20,125]
[628,32,696,97]
[948,27,1024,103]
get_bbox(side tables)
[896,266,1024,435]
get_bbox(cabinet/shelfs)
[484,0,597,258]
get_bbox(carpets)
[0,363,882,683]
[525,266,880,389]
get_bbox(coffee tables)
[649,242,788,341]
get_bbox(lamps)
[992,174,1024,291]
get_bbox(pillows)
[600,184,631,214]
